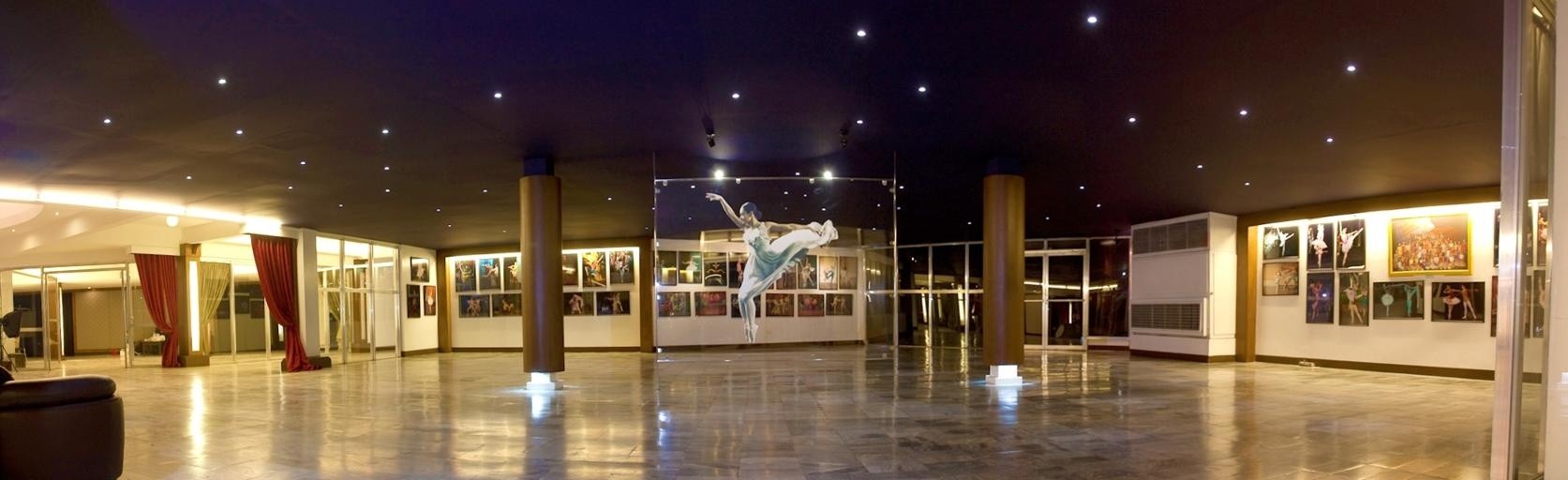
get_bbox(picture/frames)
[1388,212,1473,278]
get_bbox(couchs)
[0,366,125,480]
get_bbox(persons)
[1265,227,1295,258]
[669,190,847,344]
[1382,281,1418,317]
[1538,211,1547,265]
[1443,284,1479,320]
[1344,282,1368,324]
[1274,265,1297,294]
[410,251,631,316]
[1337,221,1364,266]
[1308,224,1327,268]
[1538,276,1545,331]
[1309,280,1325,322]
[1394,230,1467,270]
